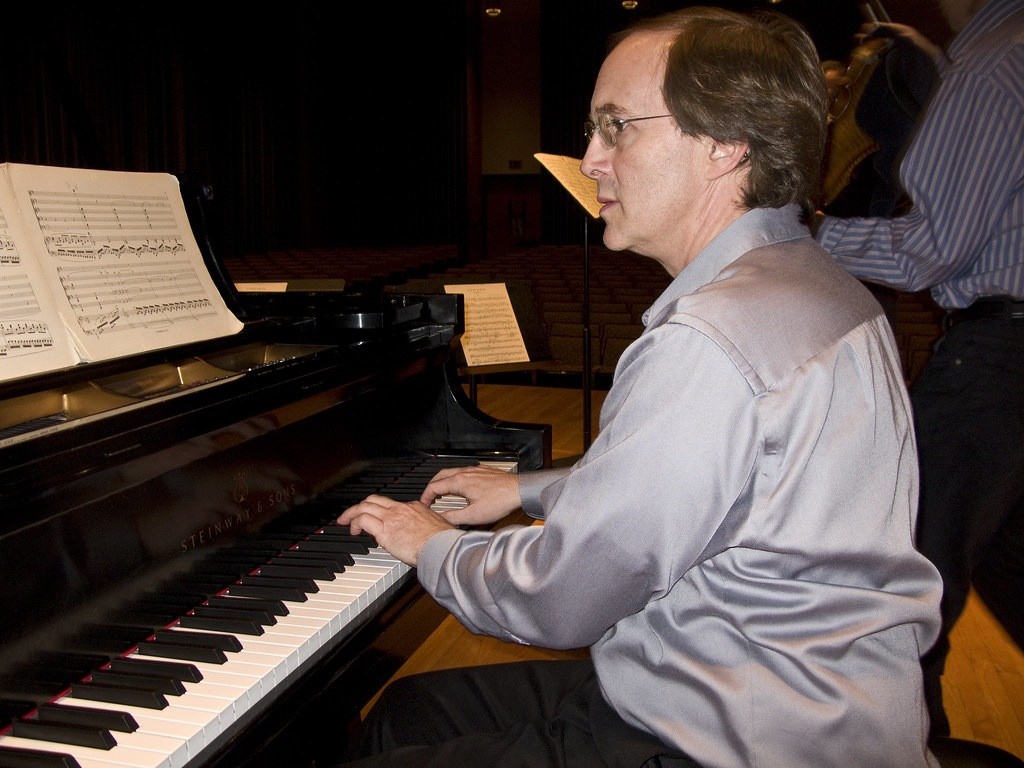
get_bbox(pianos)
[0,290,551,768]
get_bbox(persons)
[332,1,951,768]
[804,0,1024,759]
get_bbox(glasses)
[583,113,673,149]
